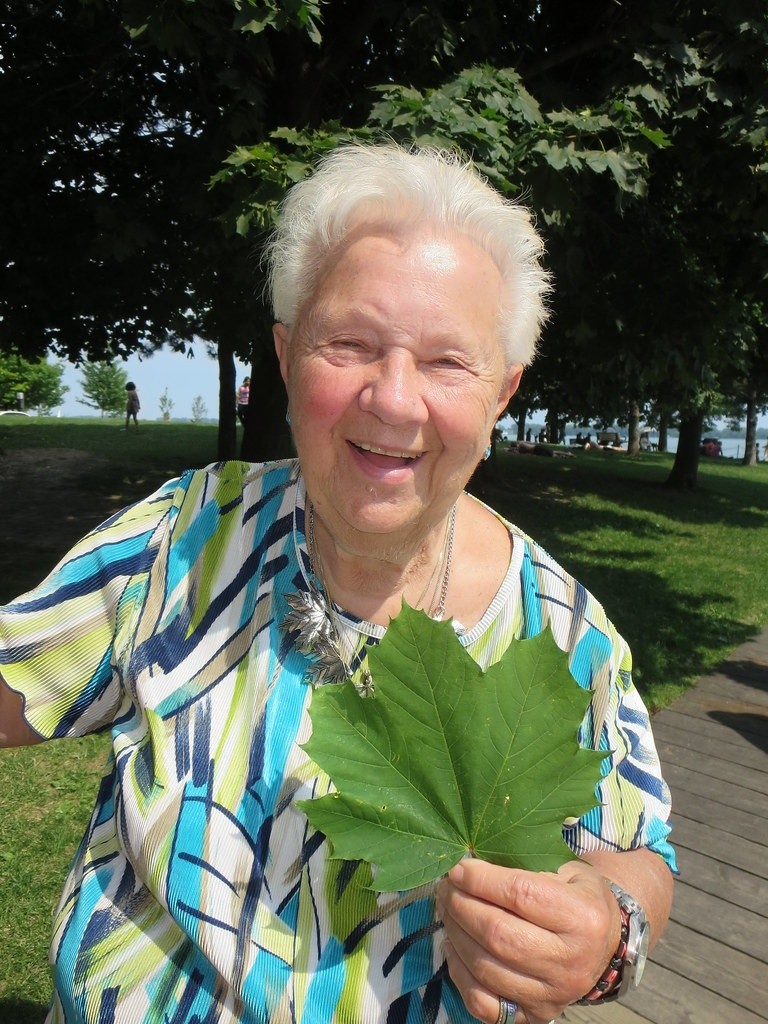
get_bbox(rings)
[496,996,517,1024]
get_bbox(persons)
[0,148,678,1024]
[237,376,250,426]
[762,436,768,463]
[640,433,652,450]
[756,443,761,460]
[526,428,532,441]
[125,381,140,432]
[511,441,574,457]
[577,432,591,445]
[539,429,546,442]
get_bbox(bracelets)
[585,912,629,1000]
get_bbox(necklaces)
[280,502,454,696]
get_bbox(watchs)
[576,881,650,1006]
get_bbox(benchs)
[641,433,658,451]
[595,431,628,447]
